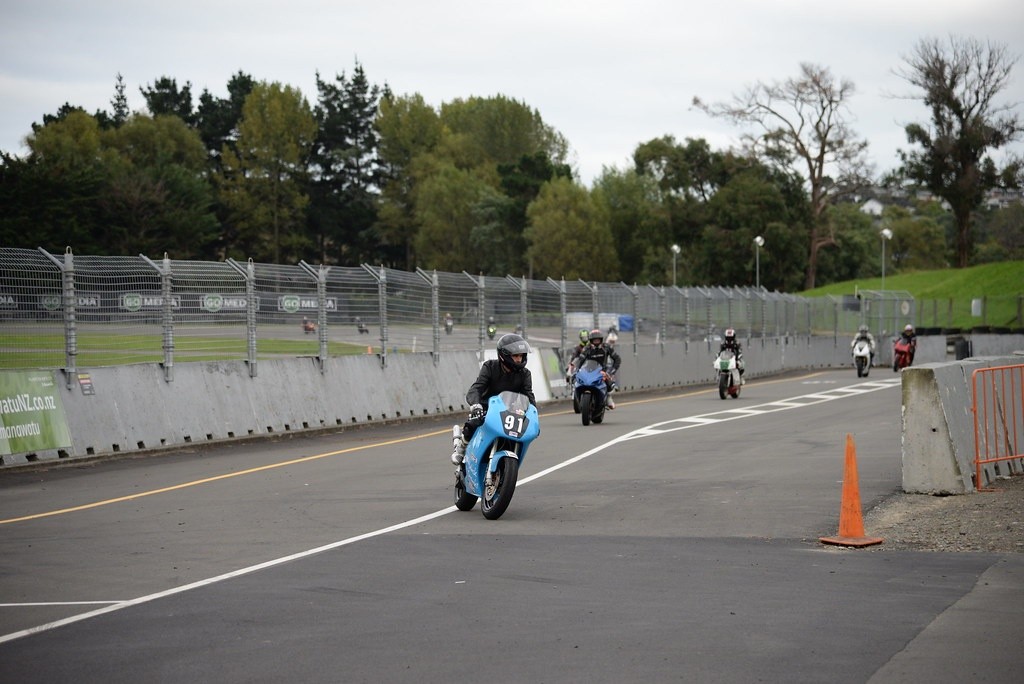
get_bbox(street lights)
[879,229,894,333]
[754,237,765,290]
[671,245,681,287]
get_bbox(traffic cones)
[818,433,884,545]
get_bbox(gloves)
[470,407,486,422]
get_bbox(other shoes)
[609,396,617,409]
[451,438,469,466]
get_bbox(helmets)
[724,327,736,341]
[579,329,592,345]
[496,333,531,374]
[858,325,870,335]
[905,324,913,334]
[588,330,605,350]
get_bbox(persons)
[604,325,618,341]
[894,323,916,366]
[849,324,876,368]
[301,316,310,334]
[355,317,363,328]
[443,312,453,331]
[706,324,721,337]
[514,325,525,341]
[451,334,537,466]
[716,329,746,385]
[486,316,496,333]
[568,330,621,410]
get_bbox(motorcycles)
[605,330,618,348]
[566,354,619,425]
[713,350,746,400]
[301,323,316,334]
[357,323,369,334]
[452,392,540,520]
[892,338,916,372]
[444,320,453,334]
[486,325,496,340]
[851,335,872,378]
